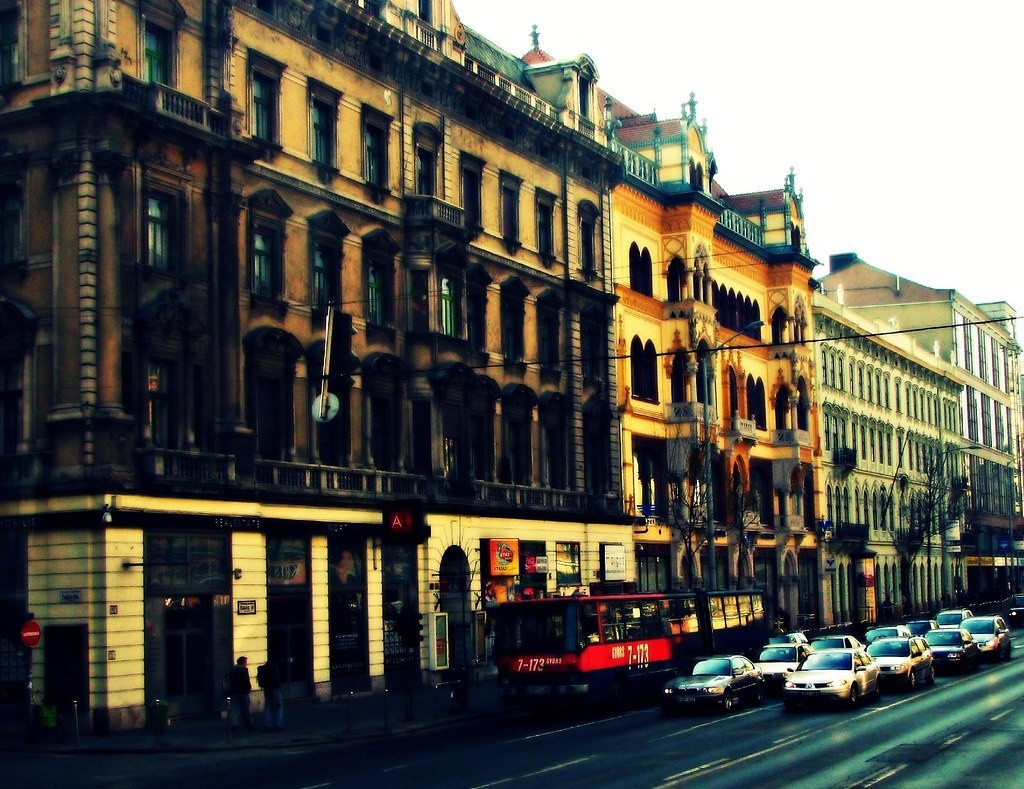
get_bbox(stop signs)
[21,621,41,646]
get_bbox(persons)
[230,656,255,732]
[257,649,285,731]
[883,597,893,622]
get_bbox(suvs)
[958,614,1012,663]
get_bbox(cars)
[807,634,865,651]
[660,653,767,717]
[782,648,883,708]
[752,642,814,684]
[864,634,935,696]
[863,609,974,649]
[922,627,980,676]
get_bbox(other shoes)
[231,725,239,732]
[245,725,253,730]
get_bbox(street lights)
[700,318,768,592]
[937,445,983,610]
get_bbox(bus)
[483,589,771,715]
